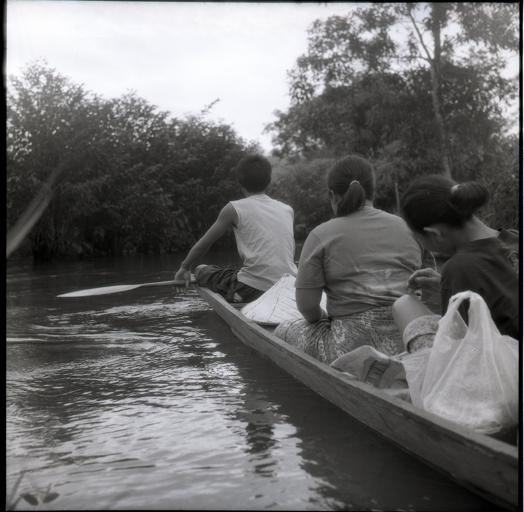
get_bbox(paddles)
[56,279,196,298]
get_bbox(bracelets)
[179,261,193,271]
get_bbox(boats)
[191,259,521,508]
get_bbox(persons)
[172,151,299,305]
[327,171,520,407]
[270,154,430,368]
